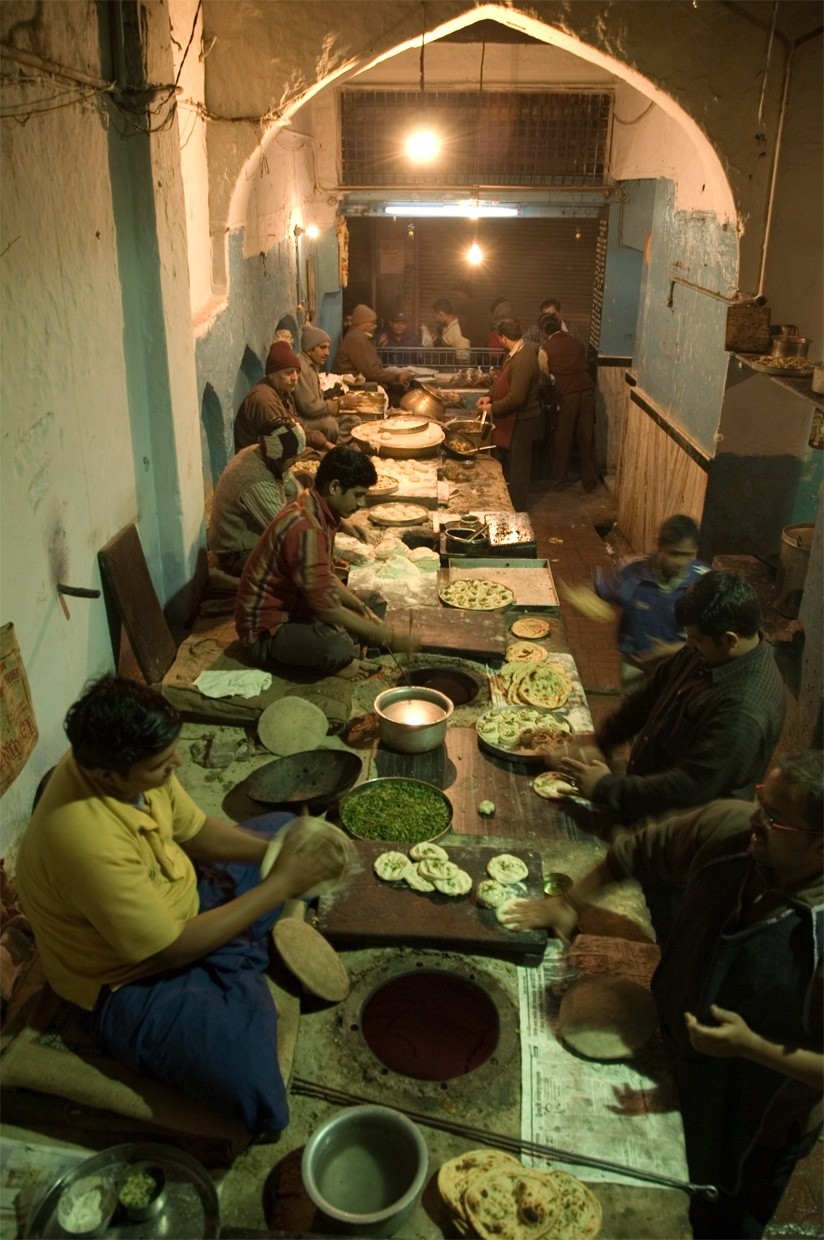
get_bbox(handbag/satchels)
[539,372,558,413]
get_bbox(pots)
[227,749,363,815]
[444,420,495,456]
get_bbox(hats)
[391,312,407,322]
[301,326,331,352]
[352,305,377,326]
[265,342,301,374]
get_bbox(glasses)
[754,785,817,837]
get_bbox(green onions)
[383,849,524,895]
[342,781,450,845]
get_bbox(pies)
[437,1149,603,1240]
[511,618,550,638]
[260,816,361,899]
[566,588,615,623]
[499,660,569,710]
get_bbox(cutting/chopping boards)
[385,608,507,660]
[484,513,535,547]
[317,840,547,952]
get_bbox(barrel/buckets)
[771,523,817,620]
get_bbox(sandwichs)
[504,641,548,663]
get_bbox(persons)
[375,312,419,366]
[426,299,471,366]
[477,297,596,512]
[332,305,412,385]
[291,322,365,420]
[209,419,376,575]
[236,446,418,671]
[234,341,340,453]
[14,675,346,1139]
[503,514,824,1240]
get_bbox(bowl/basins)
[56,1171,118,1239]
[444,528,486,554]
[543,872,572,898]
[442,430,476,459]
[302,1104,430,1240]
[118,1165,170,1221]
[374,685,454,753]
[771,335,813,360]
